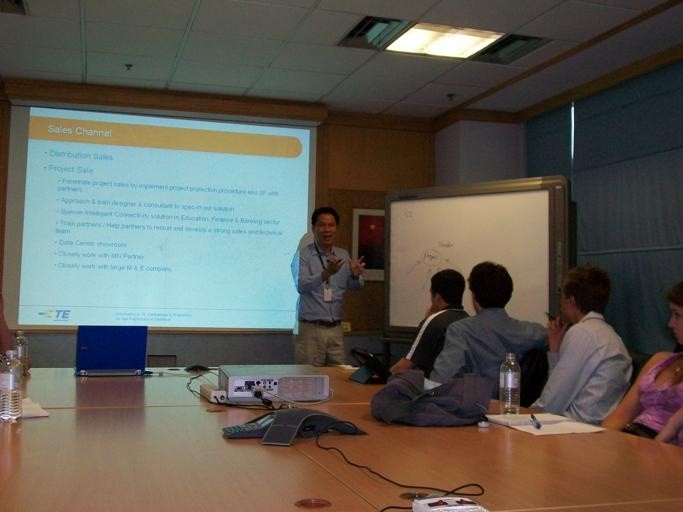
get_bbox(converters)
[201,383,228,404]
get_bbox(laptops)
[75,324,148,375]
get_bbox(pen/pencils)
[546,312,564,326]
[530,413,542,429]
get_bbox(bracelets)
[327,270,332,274]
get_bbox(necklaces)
[674,362,681,371]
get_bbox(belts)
[302,317,342,328]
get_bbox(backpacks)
[371,369,494,427]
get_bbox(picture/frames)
[352,208,386,283]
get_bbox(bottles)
[0,352,23,421]
[11,329,32,368]
[501,352,522,411]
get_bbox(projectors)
[218,364,330,403]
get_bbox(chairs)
[518,347,550,408]
[630,351,653,389]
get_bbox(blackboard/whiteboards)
[384,175,571,332]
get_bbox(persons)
[602,281,682,447]
[430,261,548,399]
[291,207,365,367]
[528,263,633,426]
[390,269,470,379]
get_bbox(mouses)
[184,364,208,372]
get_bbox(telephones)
[222,408,368,446]
